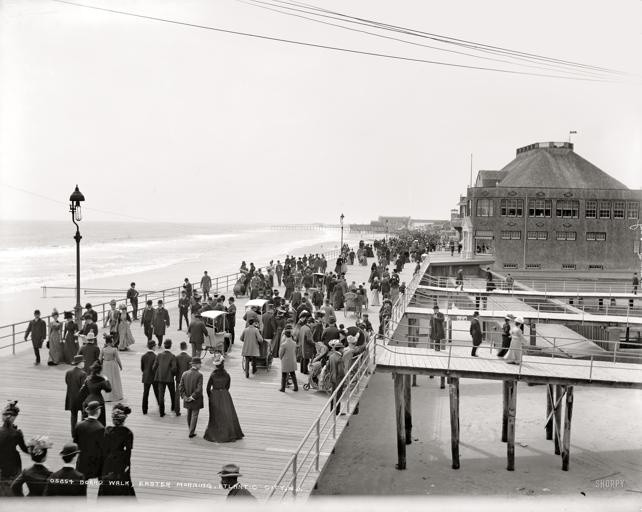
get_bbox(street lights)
[340,213,345,247]
[68,185,84,331]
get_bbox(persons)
[1,228,525,497]
[631,271,640,293]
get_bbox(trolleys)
[197,310,231,359]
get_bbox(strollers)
[303,347,332,392]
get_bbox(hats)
[29,437,52,460]
[85,401,102,413]
[111,404,130,424]
[60,443,83,456]
[218,464,240,477]
[71,355,86,364]
[189,357,201,364]
[213,354,223,365]
[90,363,101,373]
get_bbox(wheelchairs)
[241,340,272,373]
